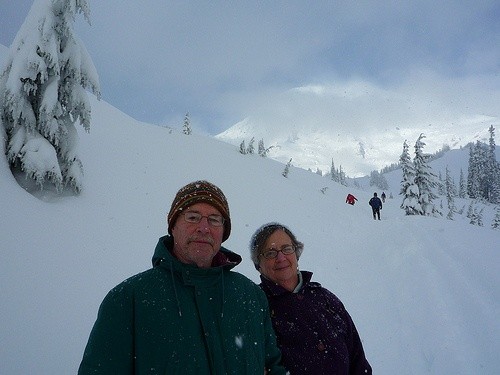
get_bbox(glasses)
[258,245,297,259]
[176,210,226,228]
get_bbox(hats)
[167,180,231,243]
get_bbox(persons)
[369,192,382,220]
[78,179,290,375]
[345,194,358,205]
[381,192,386,203]
[248,222,373,375]
